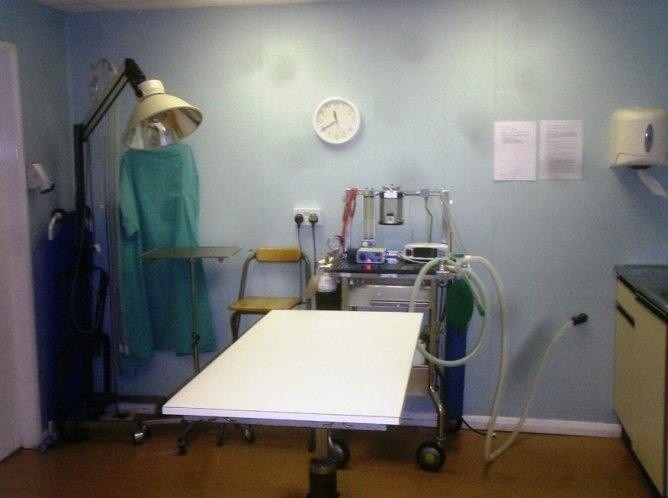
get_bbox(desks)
[130,246,256,454]
[320,256,456,470]
[160,310,425,497]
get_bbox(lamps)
[56,51,205,450]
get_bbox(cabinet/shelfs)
[612,280,667,497]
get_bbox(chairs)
[230,247,311,341]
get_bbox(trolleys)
[38,206,113,455]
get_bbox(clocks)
[313,94,360,147]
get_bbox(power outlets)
[294,208,322,226]
